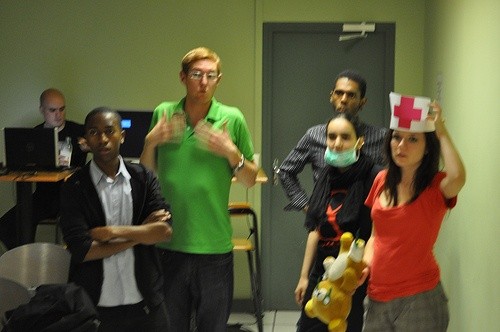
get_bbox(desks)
[0,170,268,243]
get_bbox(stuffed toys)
[304,232,369,332]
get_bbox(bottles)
[59,136,73,170]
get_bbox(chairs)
[38,213,63,245]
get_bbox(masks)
[324,136,364,168]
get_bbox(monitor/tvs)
[116,109,155,164]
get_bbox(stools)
[227,201,264,332]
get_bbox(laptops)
[2,126,68,174]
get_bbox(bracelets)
[234,154,245,172]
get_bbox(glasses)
[190,73,222,82]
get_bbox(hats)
[389,92,435,133]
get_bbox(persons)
[59,107,173,332]
[278,70,466,332]
[0,89,88,252]
[140,46,258,331]
